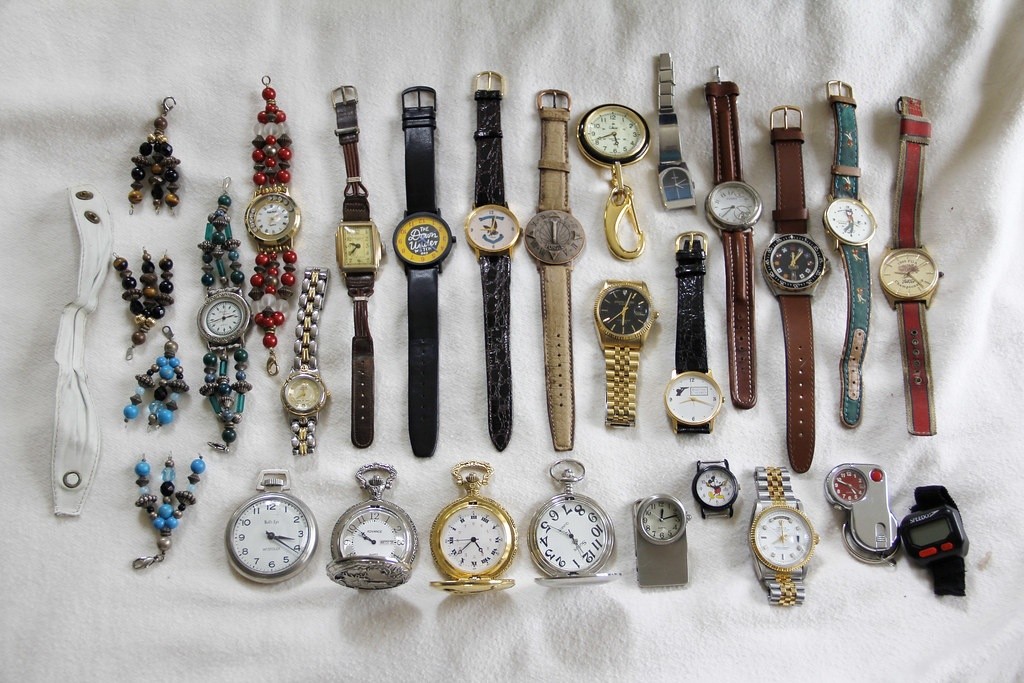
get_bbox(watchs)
[898,486,970,596]
[195,54,943,607]
